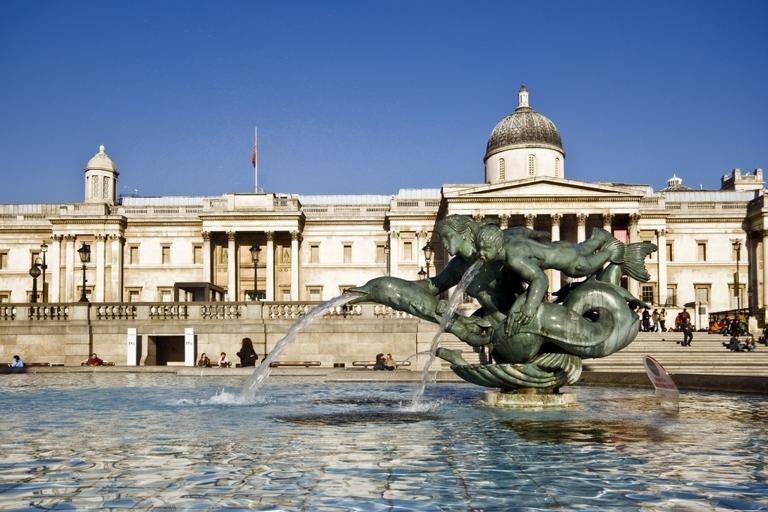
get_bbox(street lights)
[39,238,49,304]
[76,239,92,302]
[417,265,427,280]
[420,240,434,278]
[731,238,742,309]
[29,253,45,304]
[249,239,262,301]
[384,238,390,277]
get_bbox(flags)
[251,138,257,168]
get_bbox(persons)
[640,308,695,347]
[198,352,229,368]
[708,312,768,352]
[373,353,397,370]
[237,338,258,367]
[7,355,24,368]
[86,353,103,367]
[413,214,610,337]
